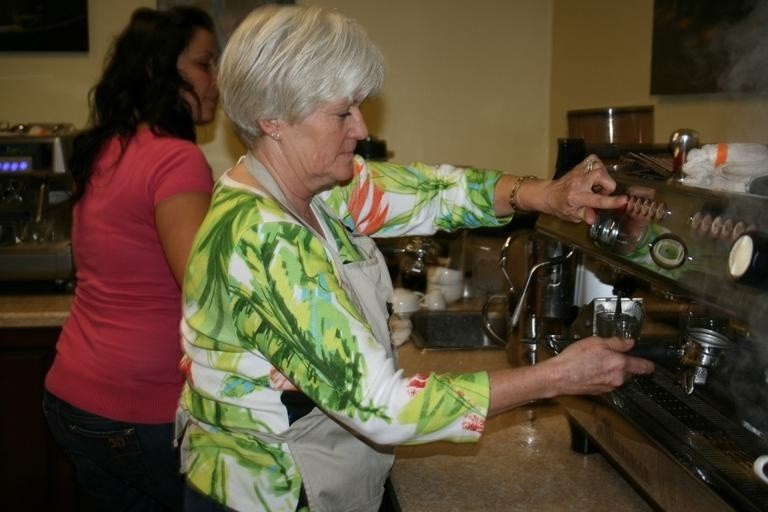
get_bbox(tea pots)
[400,237,440,291]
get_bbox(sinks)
[398,309,507,353]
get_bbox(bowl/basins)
[428,266,464,301]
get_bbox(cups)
[594,311,645,344]
[393,289,445,315]
[671,325,738,389]
